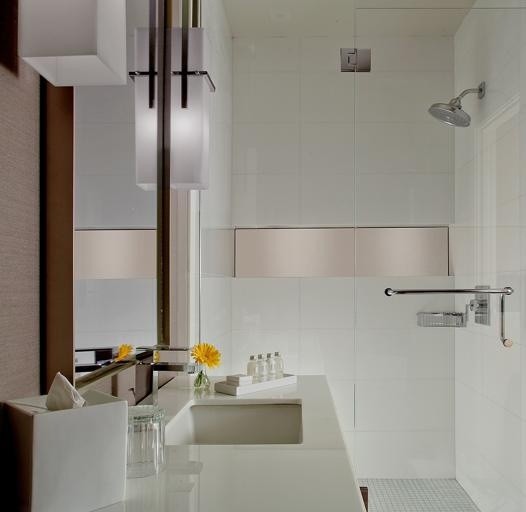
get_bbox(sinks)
[164,396,304,445]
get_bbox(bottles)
[247,351,285,383]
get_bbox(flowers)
[190,341,221,369]
[113,343,133,363]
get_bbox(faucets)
[133,361,204,405]
[74,346,119,365]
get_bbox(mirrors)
[70,0,170,384]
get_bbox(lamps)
[128,0,158,193]
[170,0,218,191]
[18,1,129,88]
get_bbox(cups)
[127,406,164,479]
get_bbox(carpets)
[365,477,478,512]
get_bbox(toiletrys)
[245,352,284,384]
